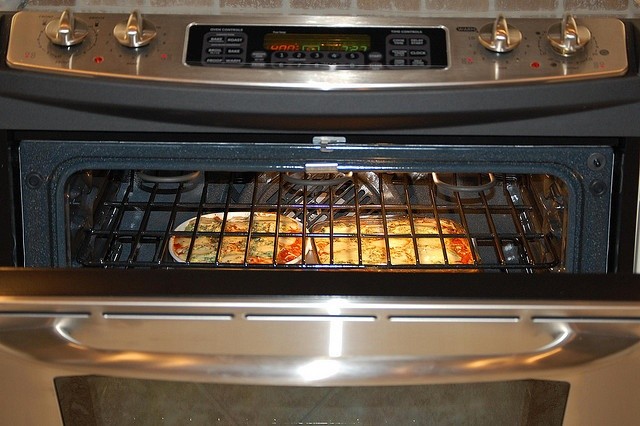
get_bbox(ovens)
[1,131,640,424]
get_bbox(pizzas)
[173,214,307,266]
[311,218,480,275]
